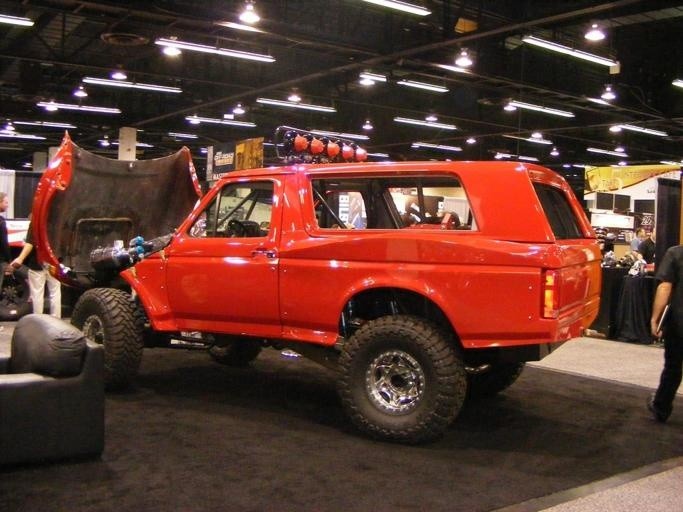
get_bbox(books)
[656,304,671,333]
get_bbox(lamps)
[584,3,605,41]
[365,0,432,17]
[155,37,277,65]
[240,2,260,23]
[0,14,35,28]
[523,35,618,68]
[1,67,683,166]
[455,3,473,67]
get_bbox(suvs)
[21,124,608,451]
[1,216,34,320]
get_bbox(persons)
[650,244,683,423]
[0,193,9,331]
[599,226,657,264]
[350,197,361,227]
[5,214,62,320]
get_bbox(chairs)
[0,313,104,463]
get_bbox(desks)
[616,276,654,345]
[582,263,628,344]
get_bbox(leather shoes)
[647,391,668,422]
